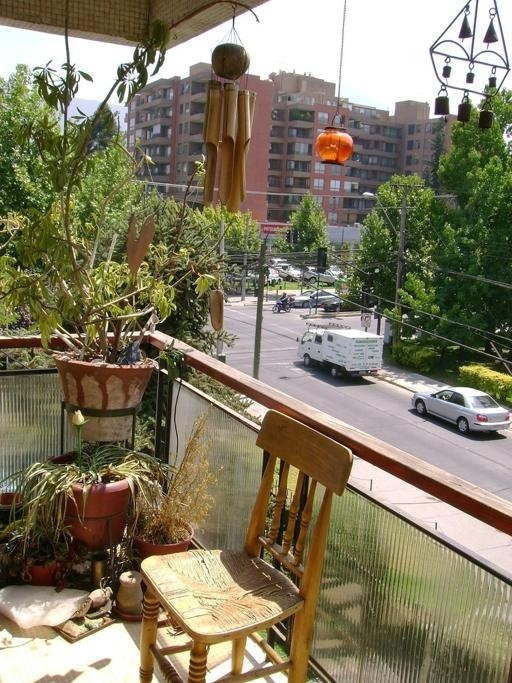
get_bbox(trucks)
[295,326,385,382]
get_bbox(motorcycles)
[272,298,292,313]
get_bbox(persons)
[279,291,289,311]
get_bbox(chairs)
[138,408,354,683]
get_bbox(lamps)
[316,0,354,164]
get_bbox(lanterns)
[314,126,353,166]
[211,42,252,82]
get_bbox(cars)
[246,256,344,285]
[410,382,512,435]
[290,285,362,311]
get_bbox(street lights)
[361,190,406,355]
